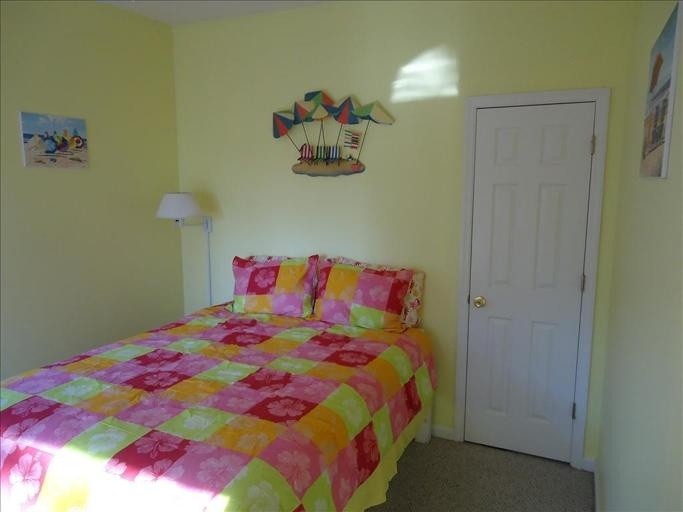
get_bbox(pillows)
[228,251,426,335]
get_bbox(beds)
[0,299,440,510]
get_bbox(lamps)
[153,191,211,232]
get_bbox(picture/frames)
[638,0,682,179]
